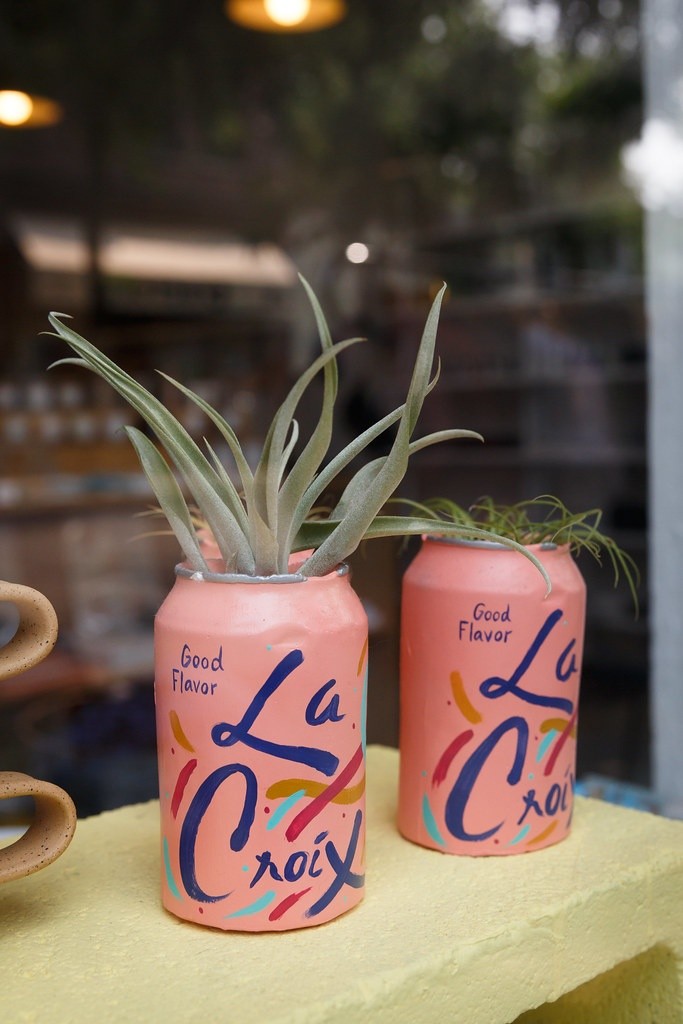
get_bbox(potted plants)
[386,494,641,856]
[42,274,553,934]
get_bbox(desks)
[0,745,683,1024]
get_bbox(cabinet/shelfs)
[374,197,649,546]
